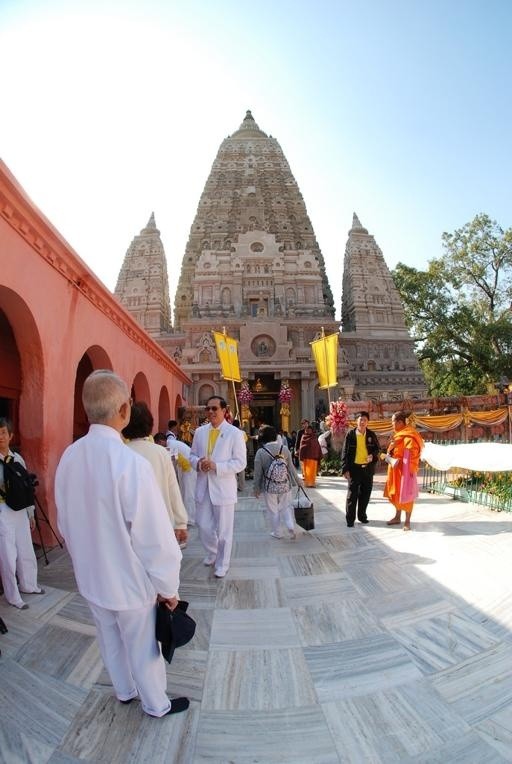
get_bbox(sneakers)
[403,520,411,531]
[347,519,355,527]
[202,556,216,566]
[148,697,193,717]
[358,516,368,523]
[387,517,400,525]
[214,566,228,579]
[120,697,134,705]
[269,528,296,540]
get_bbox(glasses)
[205,406,221,412]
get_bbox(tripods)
[31,492,63,566]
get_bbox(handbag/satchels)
[399,472,420,503]
[293,496,314,531]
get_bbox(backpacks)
[260,443,292,495]
[0,455,36,512]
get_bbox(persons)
[248,418,320,541]
[154,396,247,579]
[52,367,197,718]
[381,410,423,530]
[124,398,189,548]
[1,415,47,612]
[341,411,381,526]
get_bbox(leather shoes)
[19,585,46,595]
[8,598,29,610]
